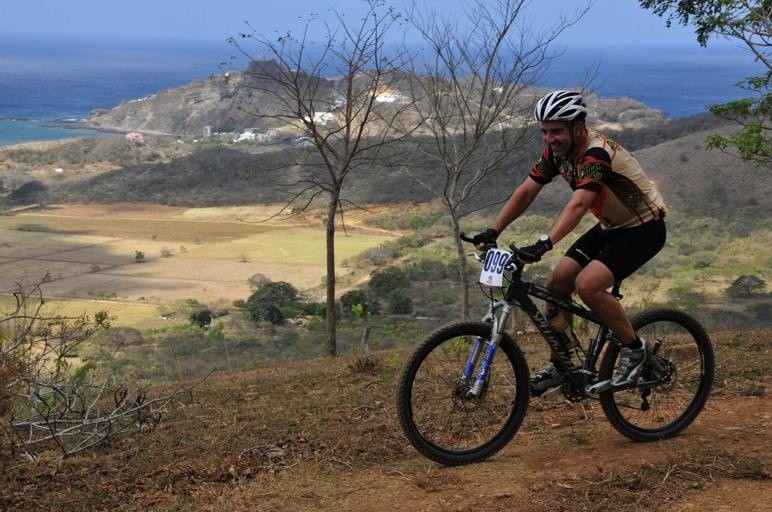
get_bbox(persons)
[473,90,668,394]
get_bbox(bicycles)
[393,234,714,467]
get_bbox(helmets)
[534,90,588,121]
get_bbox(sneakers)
[528,361,572,393]
[610,338,650,387]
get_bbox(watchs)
[540,234,553,250]
